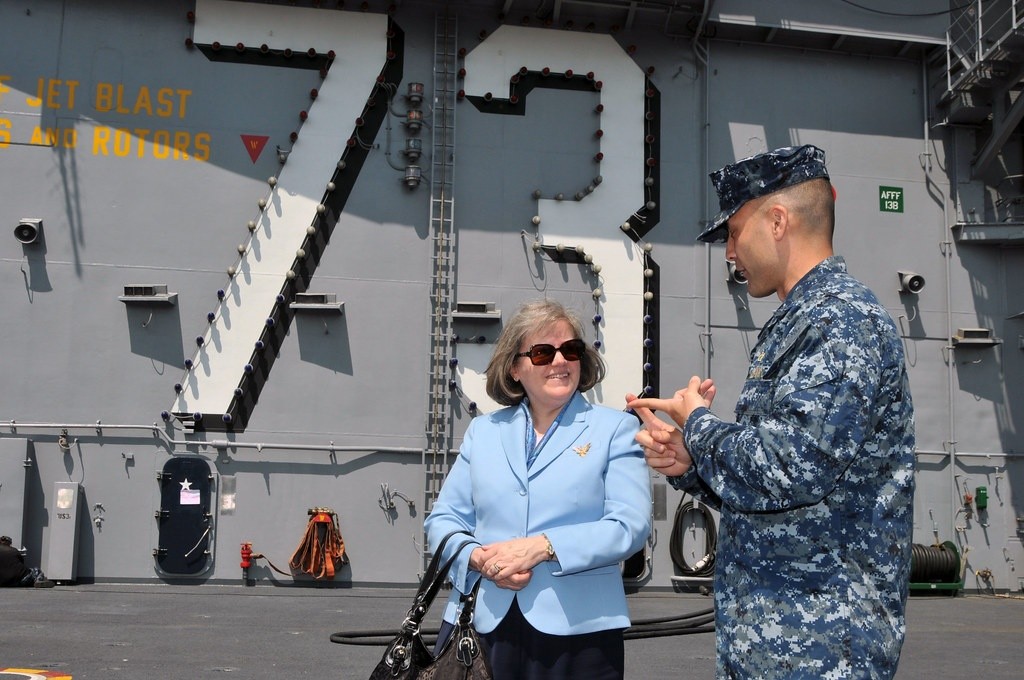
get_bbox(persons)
[625,144,918,680]
[420,299,655,680]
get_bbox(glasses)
[513,338,587,366]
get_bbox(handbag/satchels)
[368,531,493,680]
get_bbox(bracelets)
[539,532,556,563]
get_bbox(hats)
[695,146,831,243]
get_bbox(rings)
[493,563,502,572]
